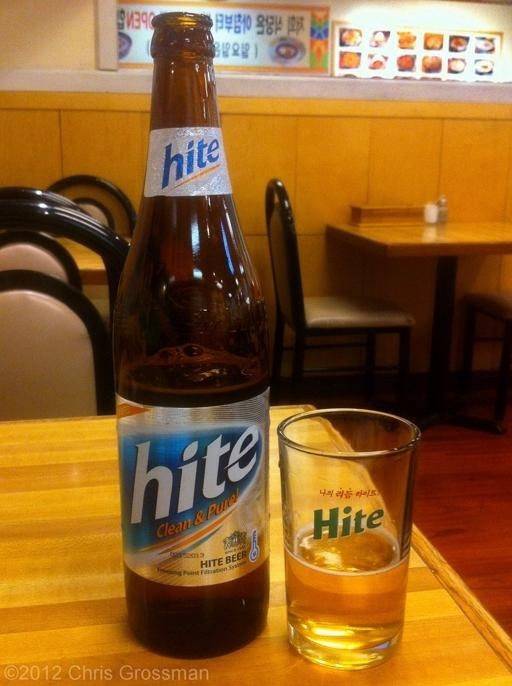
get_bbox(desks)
[1,402,512,685]
[325,218,511,426]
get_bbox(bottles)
[110,13,268,660]
[424,194,448,222]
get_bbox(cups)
[277,407,422,669]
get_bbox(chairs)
[456,287,512,421]
[0,175,137,420]
[263,177,415,431]
